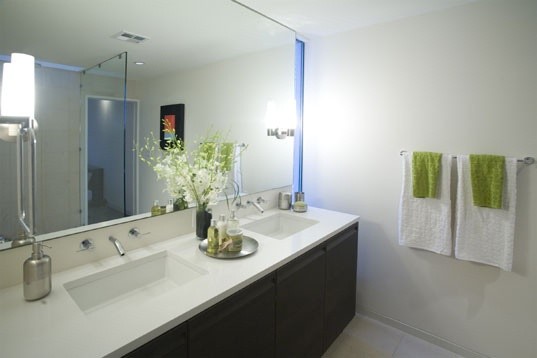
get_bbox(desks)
[88,168,107,207]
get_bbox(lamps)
[0,53,36,143]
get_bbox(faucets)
[251,201,264,214]
[107,236,127,256]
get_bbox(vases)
[173,202,187,212]
[196,212,212,240]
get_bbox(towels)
[454,154,517,274]
[413,151,442,199]
[202,141,217,168]
[219,142,233,171]
[469,154,507,210]
[218,142,242,196]
[397,151,452,257]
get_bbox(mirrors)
[0,0,295,252]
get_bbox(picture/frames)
[160,104,184,151]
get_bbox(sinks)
[239,212,318,239]
[62,249,209,329]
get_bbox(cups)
[225,227,243,252]
[294,192,305,202]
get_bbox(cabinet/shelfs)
[121,222,358,357]
